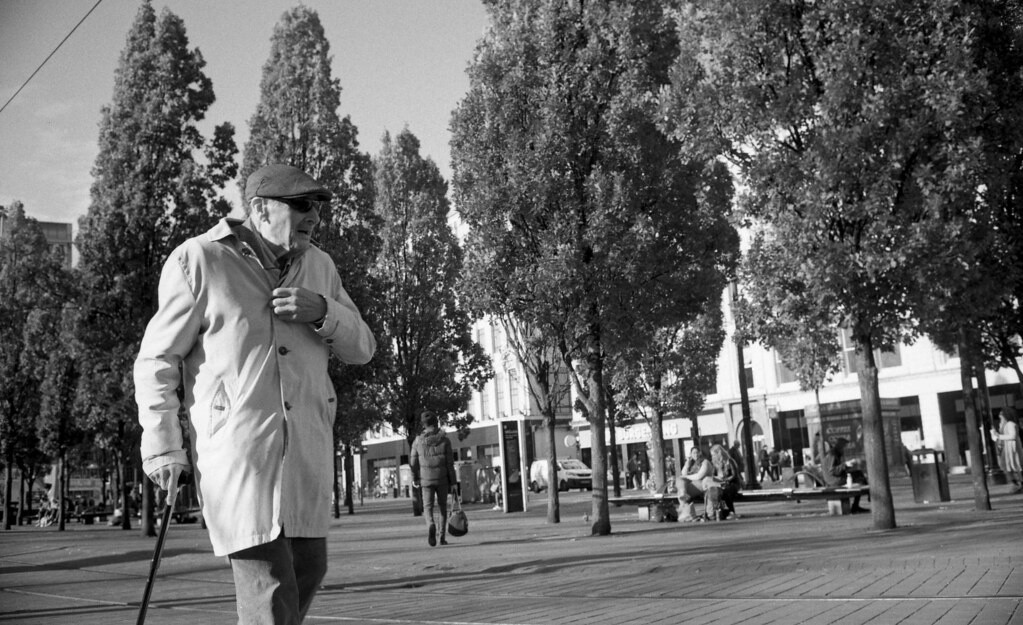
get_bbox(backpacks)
[704,487,726,519]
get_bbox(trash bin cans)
[909,445,952,503]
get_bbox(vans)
[529,459,592,492]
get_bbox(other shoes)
[493,506,502,511]
[679,495,691,500]
[440,540,448,545]
[728,511,737,519]
[851,505,871,513]
[428,524,436,547]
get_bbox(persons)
[990,407,1023,494]
[769,447,780,481]
[710,445,742,520]
[821,439,871,512]
[756,445,775,483]
[132,164,377,625]
[784,451,792,468]
[728,441,745,482]
[36,486,141,524]
[409,411,457,546]
[490,467,503,511]
[666,456,673,476]
[676,447,713,519]
[627,454,642,489]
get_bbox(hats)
[245,164,333,201]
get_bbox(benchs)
[608,485,870,521]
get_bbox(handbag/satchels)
[490,483,499,493]
[656,501,697,522]
[449,489,468,537]
[702,477,727,491]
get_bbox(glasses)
[271,198,322,215]
